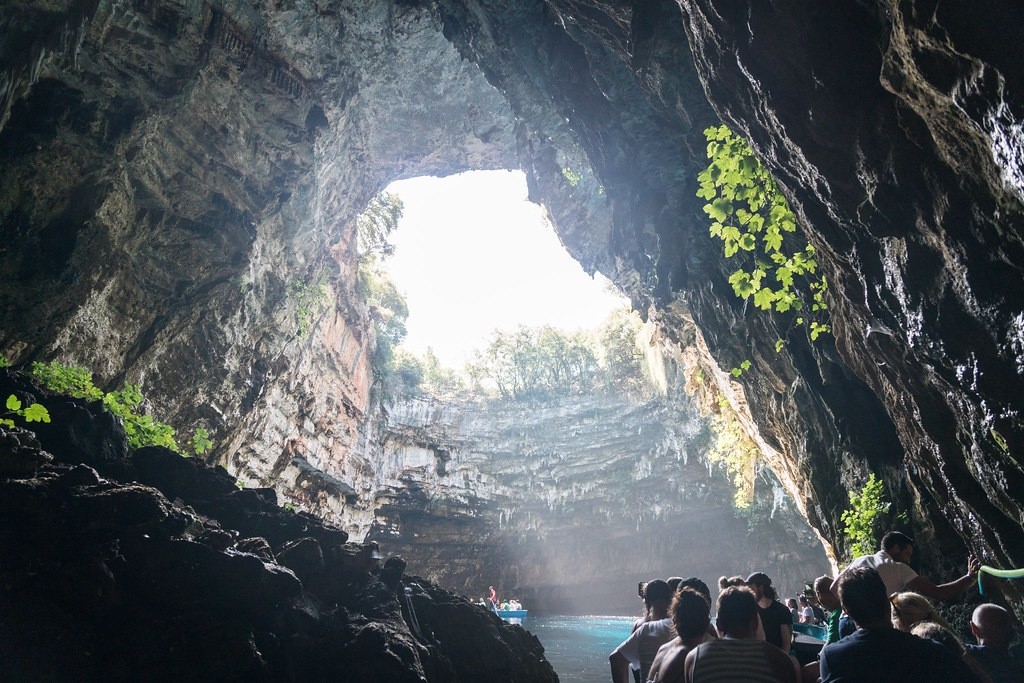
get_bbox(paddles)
[488,599,498,617]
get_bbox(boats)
[488,609,529,617]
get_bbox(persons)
[600,529,1024,683]
[468,584,526,612]
